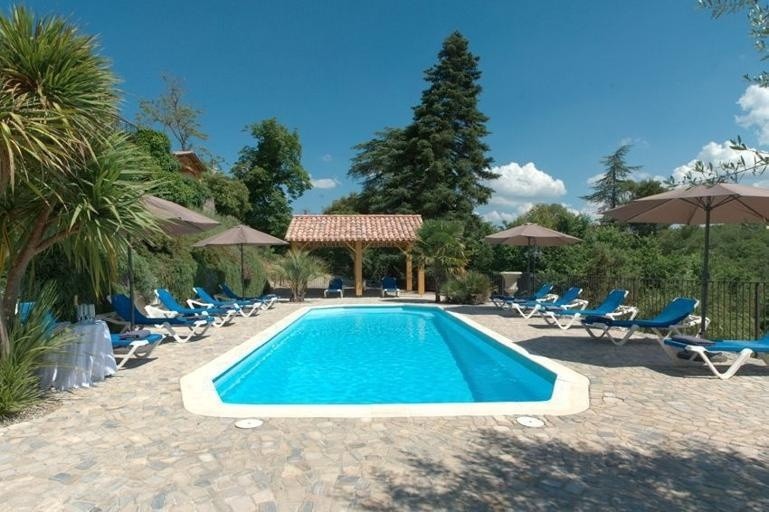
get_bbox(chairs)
[323,279,344,299]
[490,281,768,378]
[381,277,400,297]
[22,283,282,382]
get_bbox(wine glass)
[77,304,95,324]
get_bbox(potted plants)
[437,268,492,305]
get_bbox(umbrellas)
[190,222,290,301]
[594,182,769,339]
[109,194,221,333]
[480,222,583,299]
